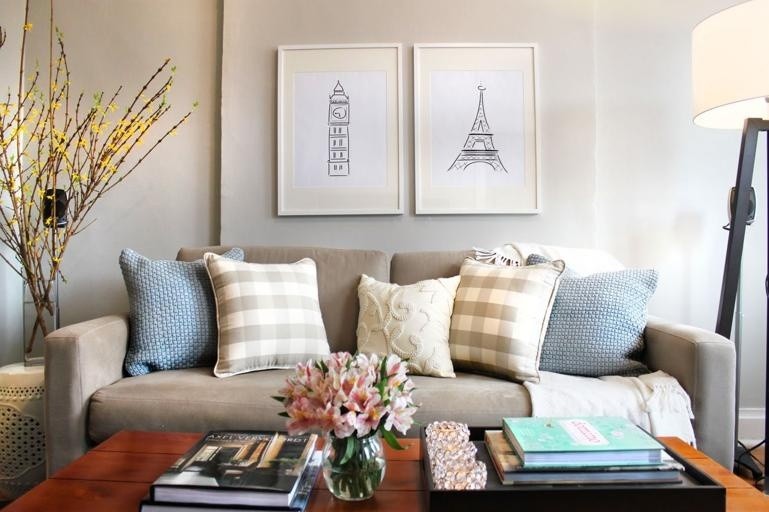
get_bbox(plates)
[419,426,726,510]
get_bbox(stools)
[0,361,45,504]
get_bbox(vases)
[20,267,60,366]
[321,431,386,502]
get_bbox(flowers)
[270,352,422,497]
[0,22,200,352]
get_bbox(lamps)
[689,1,769,131]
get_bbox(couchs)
[45,244,736,475]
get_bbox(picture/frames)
[275,43,405,218]
[412,41,541,216]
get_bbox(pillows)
[116,245,244,376]
[355,273,462,378]
[525,252,659,377]
[448,256,565,385]
[203,252,331,378]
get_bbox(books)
[483,414,684,486]
[139,426,321,511]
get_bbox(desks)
[0,428,769,512]
